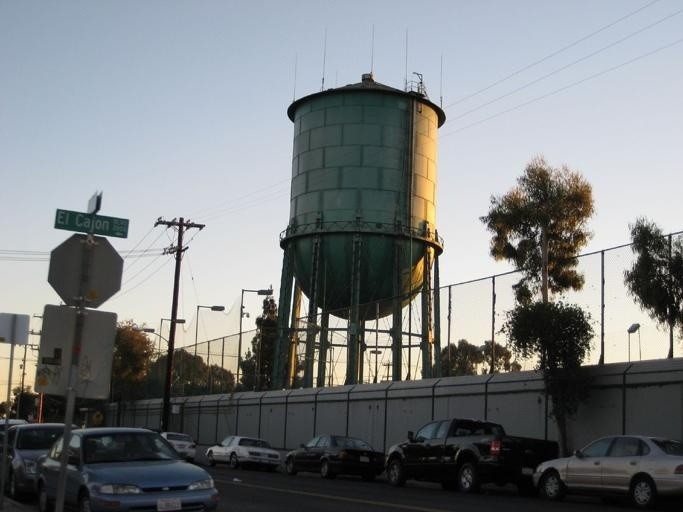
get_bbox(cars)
[35,427,219,512]
[206,435,282,470]
[155,431,197,462]
[0,418,81,502]
[532,435,683,509]
[284,434,385,481]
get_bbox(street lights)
[236,288,274,392]
[627,323,641,362]
[155,317,186,397]
[368,349,383,383]
[191,305,225,395]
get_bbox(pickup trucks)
[385,417,560,493]
